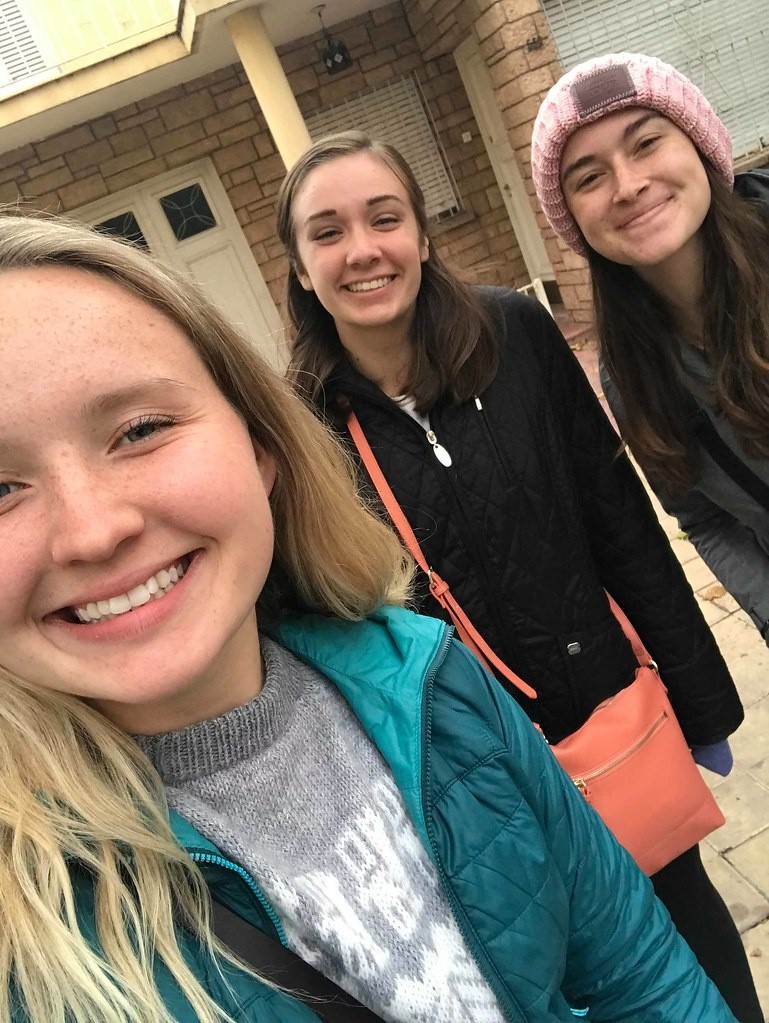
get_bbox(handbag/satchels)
[527,658,725,878]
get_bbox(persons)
[529,51,769,647]
[0,203,735,1022]
[274,132,765,1023]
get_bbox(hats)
[530,50,734,259]
